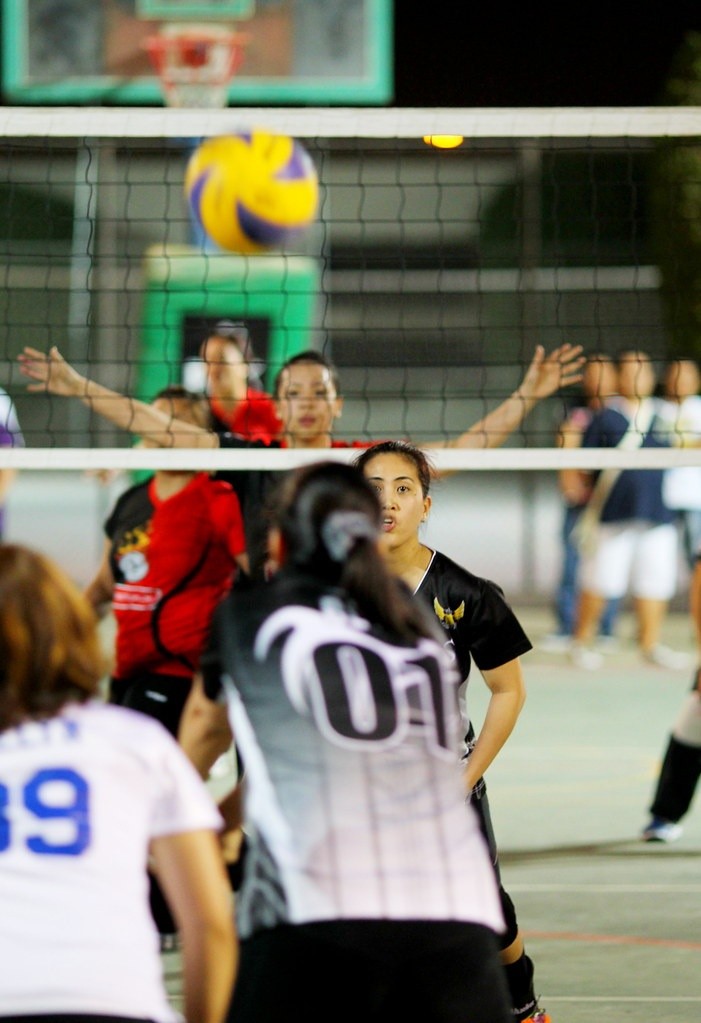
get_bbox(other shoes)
[643,821,674,841]
[640,639,680,670]
[567,642,600,671]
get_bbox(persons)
[547,349,701,843]
[0,318,587,1023]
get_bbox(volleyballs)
[183,132,319,251]
